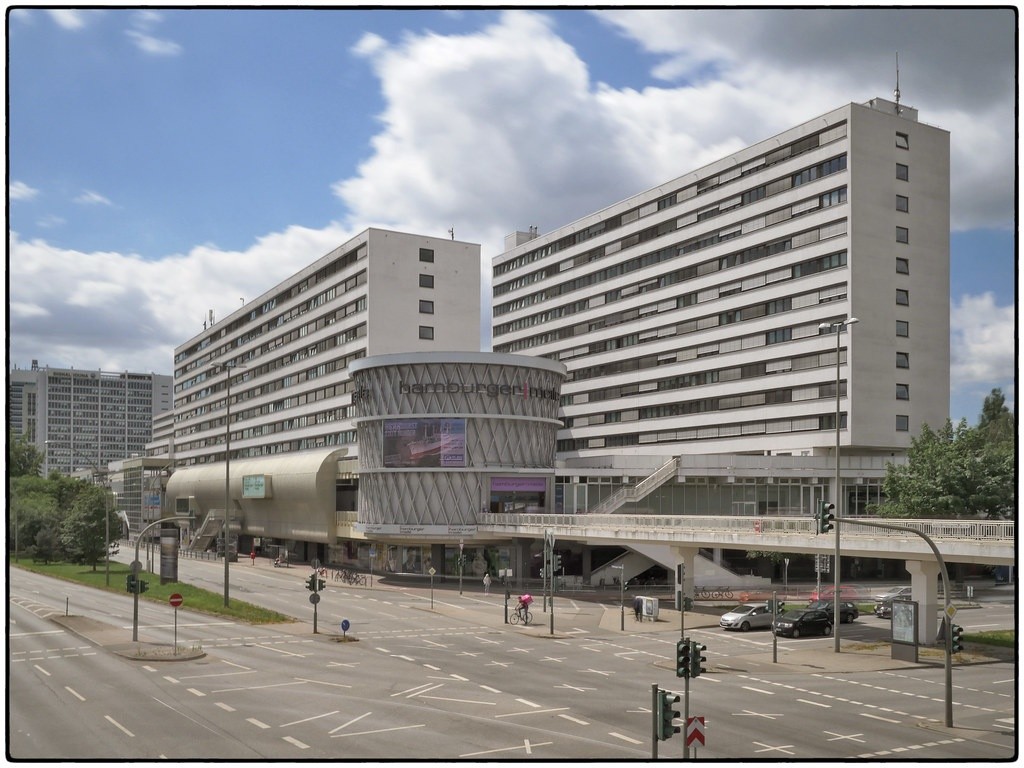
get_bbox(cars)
[718,602,774,632]
[808,585,858,603]
[873,598,905,617]
[771,608,833,639]
[804,600,860,624]
[873,586,912,602]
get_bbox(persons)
[471,547,491,577]
[634,598,643,622]
[515,597,528,625]
[483,574,491,595]
[403,547,416,573]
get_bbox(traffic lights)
[458,558,463,566]
[689,641,707,678]
[657,691,682,740]
[539,568,544,579]
[552,554,562,571]
[318,578,326,591]
[952,623,964,656]
[682,596,694,611]
[677,641,691,679]
[816,499,836,535]
[130,581,137,594]
[139,580,151,593]
[306,574,315,591]
[775,600,785,615]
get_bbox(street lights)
[818,316,861,651]
[44,440,138,586]
[611,563,625,632]
[144,456,195,574]
[210,360,247,608]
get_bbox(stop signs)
[169,593,183,606]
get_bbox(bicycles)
[711,585,733,599]
[695,585,711,598]
[509,608,533,625]
[335,568,366,586]
